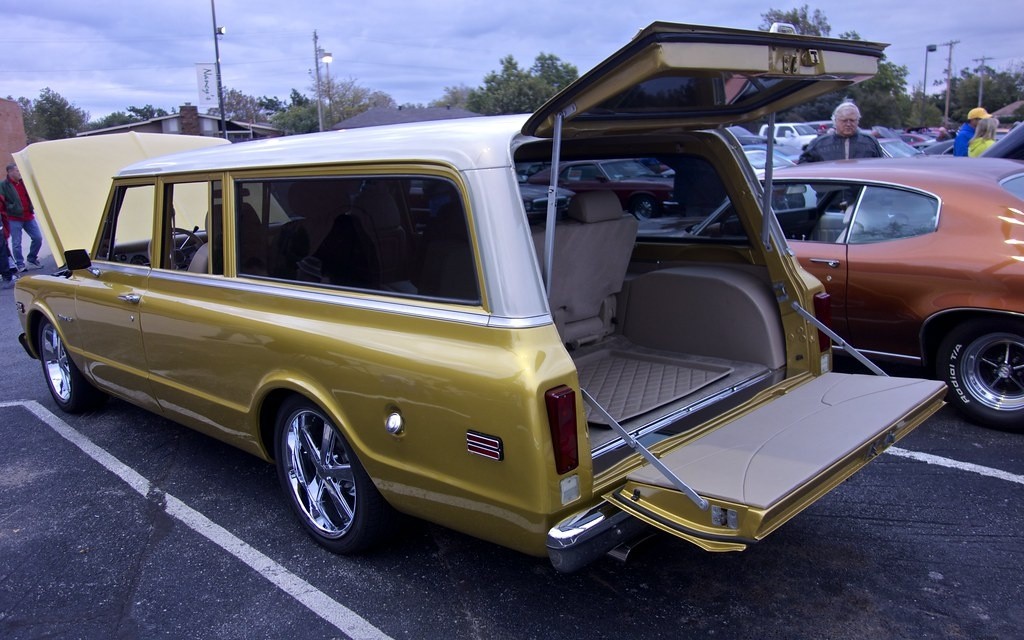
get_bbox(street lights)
[921,44,936,127]
[312,29,332,132]
[213,27,227,138]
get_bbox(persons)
[953,107,994,156]
[798,101,884,207]
[0,162,44,273]
[967,118,999,157]
[936,127,952,142]
[871,128,885,138]
[0,183,19,289]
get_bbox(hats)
[967,108,994,120]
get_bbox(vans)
[759,124,820,151]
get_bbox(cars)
[665,143,807,208]
[805,121,862,132]
[636,155,1024,431]
[408,182,576,222]
[876,138,920,159]
[12,20,949,575]
[899,132,936,151]
[921,121,1024,161]
[527,159,682,221]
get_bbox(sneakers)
[18,266,28,272]
[27,260,43,268]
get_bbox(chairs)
[350,187,407,274]
[888,194,935,238]
[837,202,889,243]
[188,204,269,273]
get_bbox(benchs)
[431,195,637,311]
